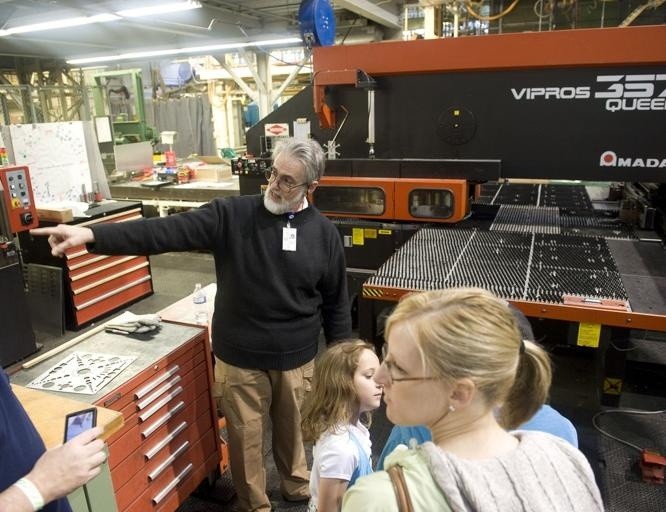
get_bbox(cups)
[232,158,238,170]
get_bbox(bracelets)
[13,477,45,510]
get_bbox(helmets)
[381,342,446,385]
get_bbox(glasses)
[264,166,308,194]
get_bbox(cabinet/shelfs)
[7,311,222,512]
[19,197,154,332]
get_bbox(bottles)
[238,156,248,167]
[152,151,177,184]
[193,283,209,327]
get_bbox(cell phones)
[64,407,97,445]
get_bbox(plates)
[141,180,172,186]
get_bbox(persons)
[26,136,357,512]
[339,286,609,512]
[298,336,384,512]
[373,302,583,475]
[0,362,110,511]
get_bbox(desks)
[9,383,124,512]
[110,173,241,216]
[152,282,231,477]
